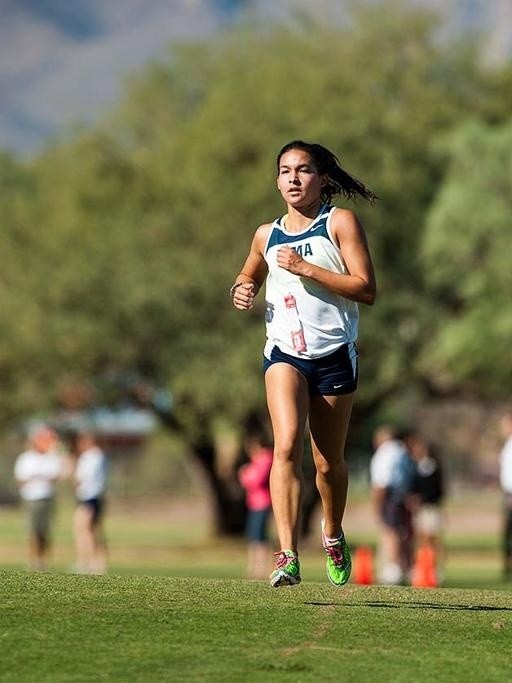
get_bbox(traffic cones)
[412,549,437,586]
[352,547,373,584]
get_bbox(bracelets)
[229,282,242,299]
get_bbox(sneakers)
[269,549,301,588]
[320,518,353,589]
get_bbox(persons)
[405,438,452,589]
[366,421,417,585]
[228,141,382,588]
[67,429,111,576]
[239,433,274,580]
[15,422,69,573]
[493,415,511,586]
